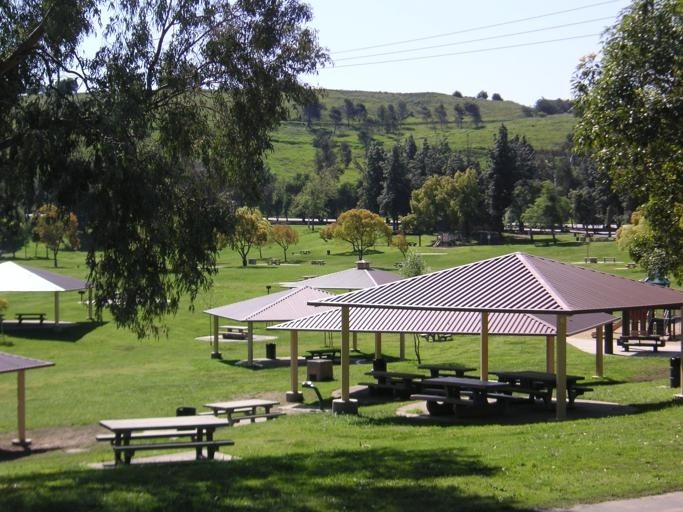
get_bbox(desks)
[202,399,280,426]
[364,364,585,411]
[98,415,229,464]
[306,349,341,356]
[220,326,248,339]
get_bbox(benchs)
[358,374,594,419]
[303,354,341,360]
[96,430,234,466]
[199,408,286,423]
[218,331,248,339]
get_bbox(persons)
[373,352,392,385]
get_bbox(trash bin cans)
[266,343,275,359]
[327,250,330,255]
[670,357,680,387]
